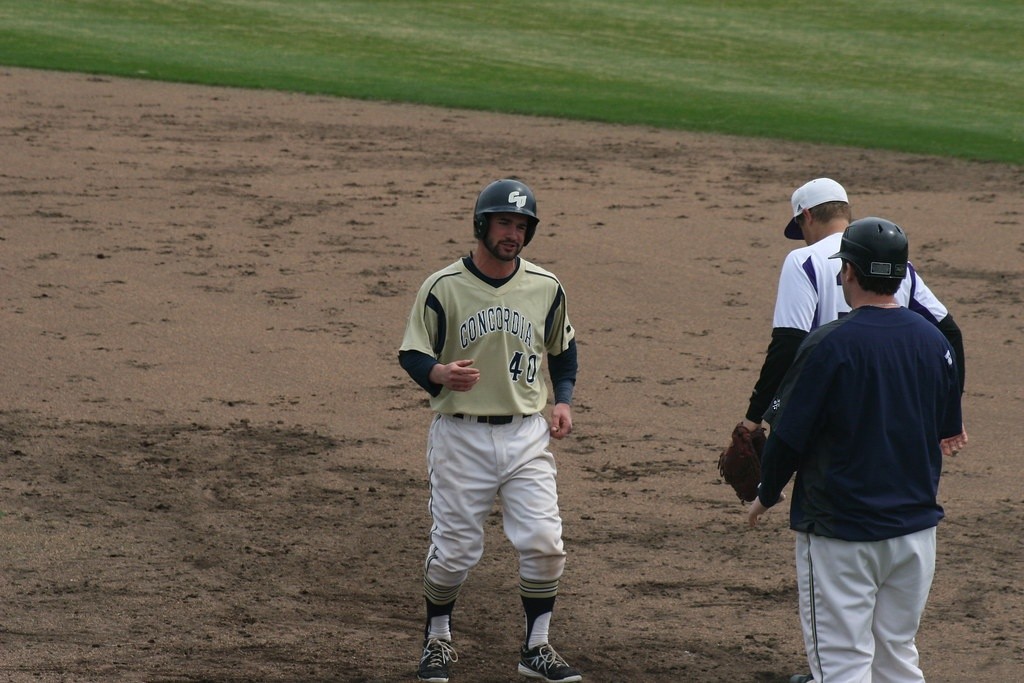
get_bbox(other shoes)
[790,674,817,683]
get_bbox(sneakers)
[518,644,582,683]
[417,637,458,683]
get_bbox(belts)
[453,413,532,424]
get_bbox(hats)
[784,178,848,240]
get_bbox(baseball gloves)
[718,420,768,501]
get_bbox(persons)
[397,178,584,683]
[748,216,963,683]
[717,177,969,502]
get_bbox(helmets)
[828,217,909,278]
[474,178,540,246]
